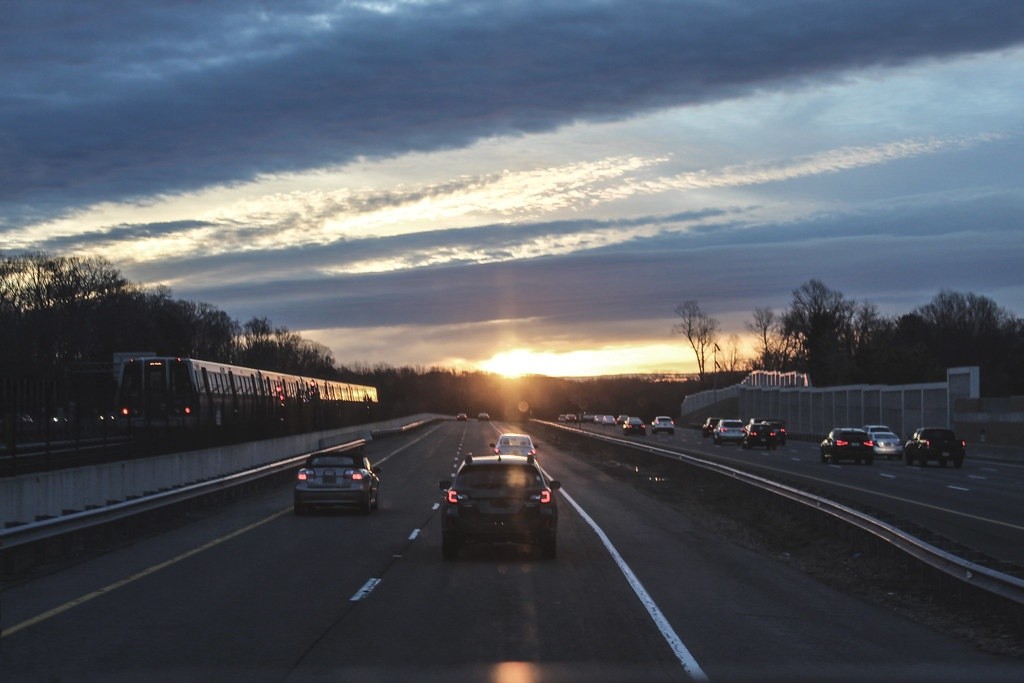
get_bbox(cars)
[863,424,891,433]
[457,413,467,421]
[869,431,903,460]
[749,418,786,446]
[566,413,577,423]
[820,427,874,465]
[651,415,675,434]
[742,423,777,450]
[622,416,646,436]
[478,412,489,421]
[617,415,628,424]
[557,414,566,422]
[293,451,383,514]
[602,415,616,426]
[593,415,603,423]
[490,433,538,456]
[702,416,722,437]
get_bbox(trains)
[115,356,378,424]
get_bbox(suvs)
[712,419,747,445]
[439,454,561,559]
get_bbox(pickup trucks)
[904,427,966,468]
